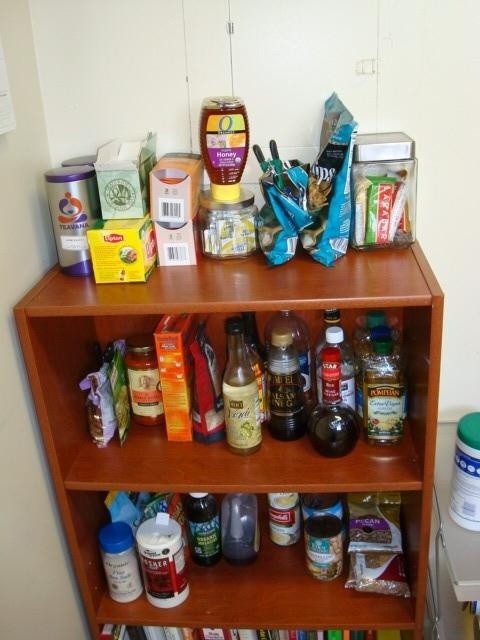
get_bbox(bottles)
[43,96,419,277]
[98,493,346,609]
[447,411,480,533]
[122,309,408,457]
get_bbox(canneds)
[303,512,345,581]
[298,497,344,521]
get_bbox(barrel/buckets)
[220,492,262,564]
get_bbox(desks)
[434,422,480,638]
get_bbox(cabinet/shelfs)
[13,239,444,640]
